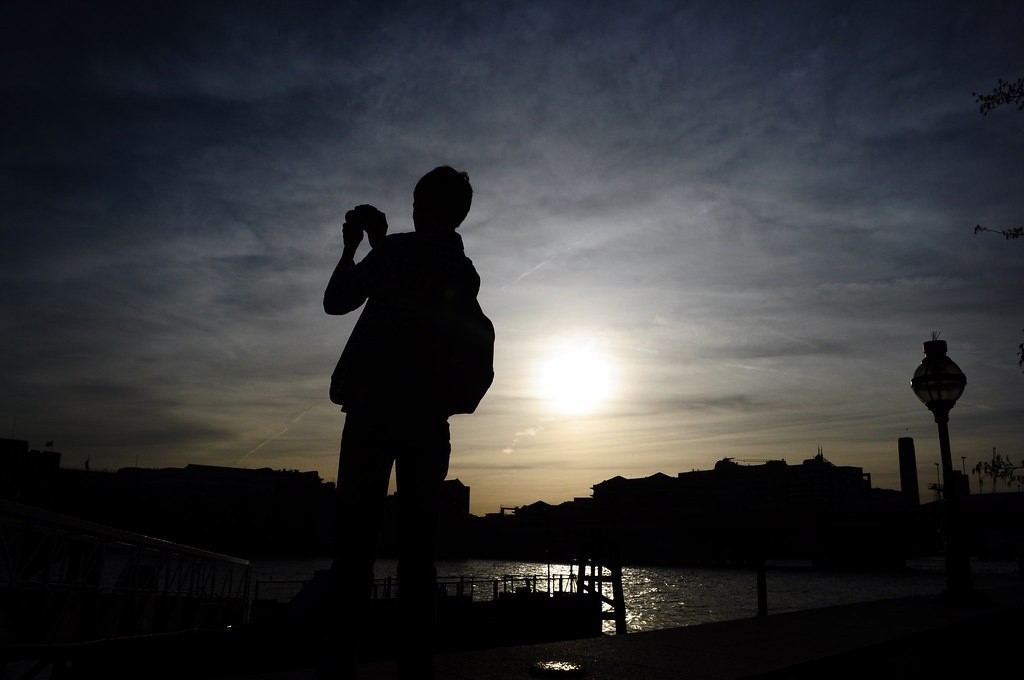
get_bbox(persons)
[314,166,478,680]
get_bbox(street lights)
[909,332,968,488]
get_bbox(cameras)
[352,205,380,231]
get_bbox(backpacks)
[382,234,496,414]
